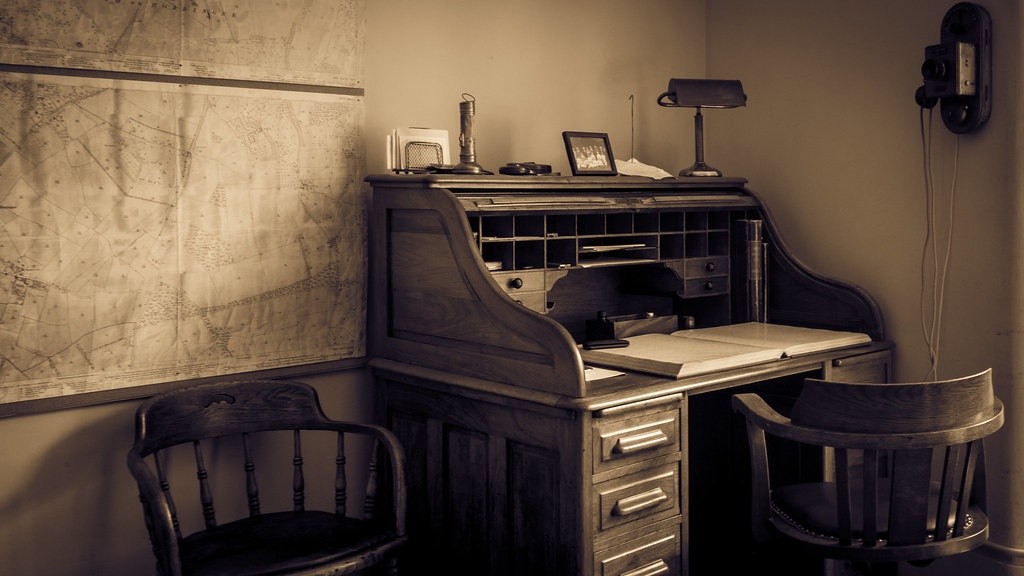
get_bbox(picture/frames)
[562,131,619,176]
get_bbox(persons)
[574,145,609,170]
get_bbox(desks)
[371,340,901,576]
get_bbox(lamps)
[658,78,749,177]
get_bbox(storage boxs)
[583,310,678,341]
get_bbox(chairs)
[732,367,1005,576]
[126,379,410,576]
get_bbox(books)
[578,320,873,379]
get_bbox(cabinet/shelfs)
[365,171,887,397]
